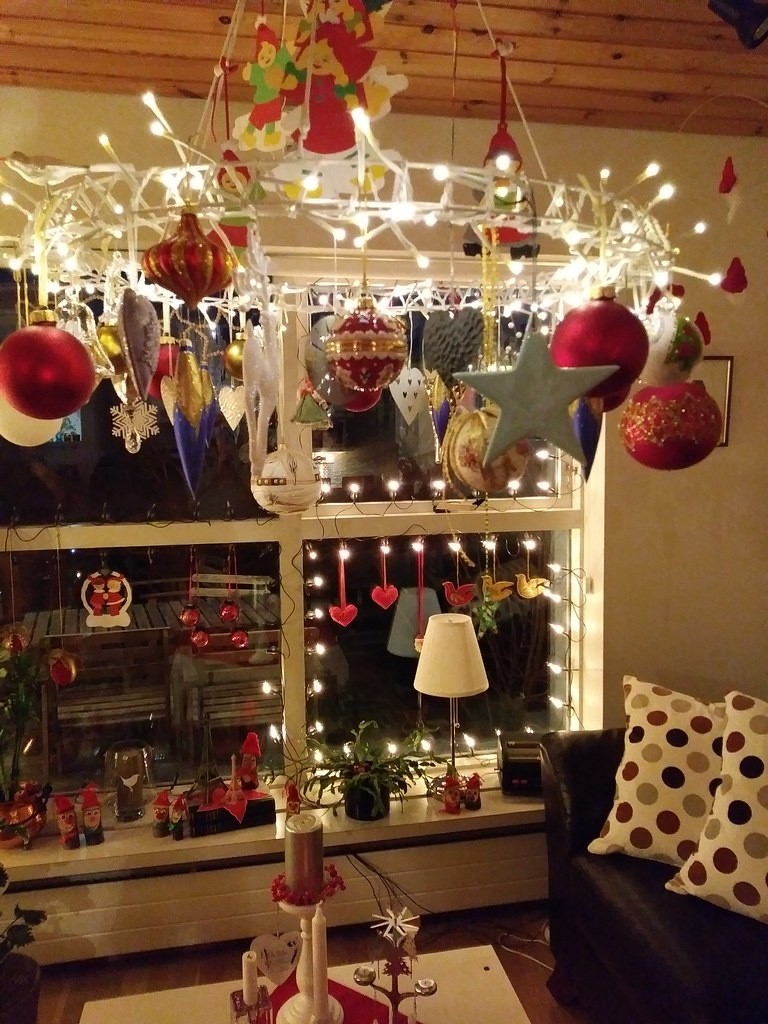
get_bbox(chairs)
[190,627,319,768]
[39,627,185,790]
[190,574,273,598]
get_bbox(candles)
[283,812,323,889]
[312,899,329,1016]
[241,950,258,1006]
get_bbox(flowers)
[271,864,346,906]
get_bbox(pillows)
[587,674,727,867]
[664,690,768,924]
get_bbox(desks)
[22,594,281,785]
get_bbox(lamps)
[412,613,490,804]
[387,586,441,727]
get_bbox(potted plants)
[303,720,460,821]
[0,862,48,1024]
[0,648,47,850]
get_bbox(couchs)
[539,729,768,1024]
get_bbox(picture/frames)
[691,355,734,447]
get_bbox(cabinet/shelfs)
[0,239,587,966]
